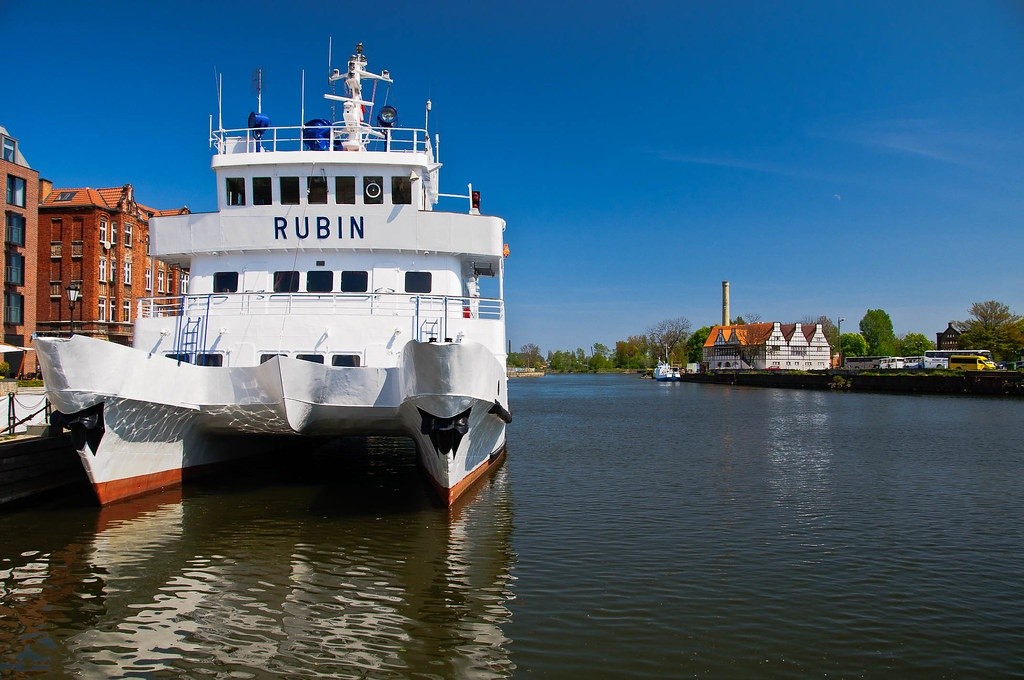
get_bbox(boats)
[33,36,514,508]
[652,364,681,383]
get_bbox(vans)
[949,355,997,371]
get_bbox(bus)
[844,356,892,371]
[877,356,923,370]
[924,350,991,371]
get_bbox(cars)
[763,366,780,371]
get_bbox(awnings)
[0,341,34,379]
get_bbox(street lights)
[64,281,82,339]
[915,341,923,370]
[837,316,845,369]
